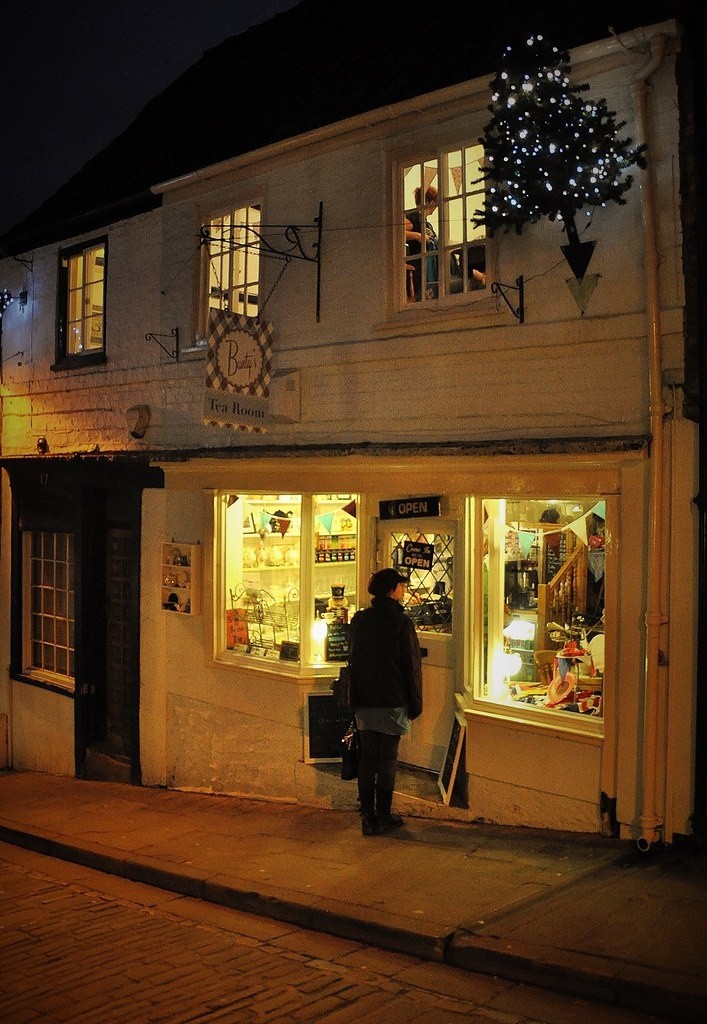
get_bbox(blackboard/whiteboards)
[324,623,351,662]
[437,712,468,806]
[303,690,354,766]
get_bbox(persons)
[404,185,438,303]
[528,510,573,574]
[350,568,423,836]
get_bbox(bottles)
[244,543,299,568]
[506,549,538,571]
[327,584,349,625]
[315,544,357,562]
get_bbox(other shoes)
[361,812,403,836]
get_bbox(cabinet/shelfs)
[244,561,356,612]
[160,541,202,616]
[230,588,299,653]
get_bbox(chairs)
[533,650,560,684]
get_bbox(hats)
[548,673,574,704]
[368,568,408,597]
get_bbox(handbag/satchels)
[340,722,364,780]
